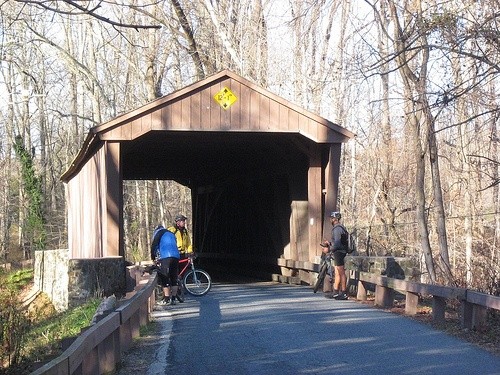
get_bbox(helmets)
[154,224,163,229]
[330,211,342,220]
[174,215,187,221]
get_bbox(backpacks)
[334,224,354,254]
[141,265,160,273]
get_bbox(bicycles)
[313,241,347,293]
[158,252,212,297]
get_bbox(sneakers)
[157,298,178,306]
[325,292,339,299]
[335,292,348,300]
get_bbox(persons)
[167,215,193,295]
[151,224,180,305]
[323,212,347,300]
